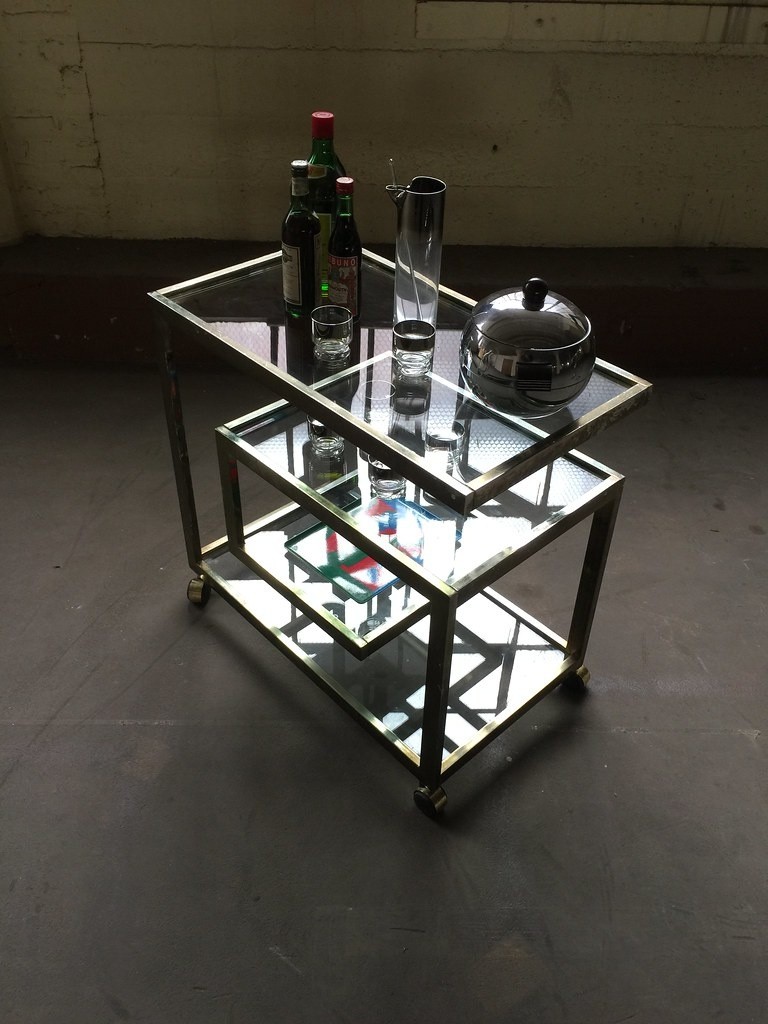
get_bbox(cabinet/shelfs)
[143,247,654,826]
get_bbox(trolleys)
[143,245,653,820]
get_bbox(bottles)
[308,111,346,297]
[282,160,322,318]
[328,177,361,324]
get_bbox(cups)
[311,305,353,350]
[307,414,343,450]
[368,454,406,491]
[392,320,436,364]
[313,346,352,400]
[391,363,432,415]
[425,427,466,470]
[359,380,396,427]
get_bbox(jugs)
[386,176,446,327]
